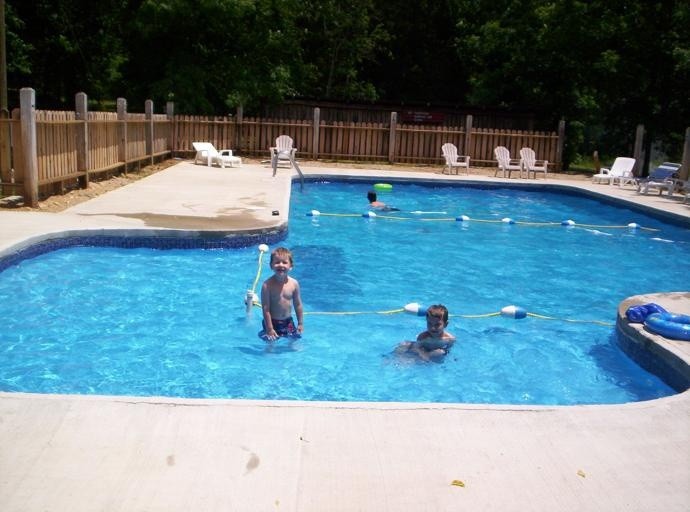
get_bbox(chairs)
[192,142,241,168]
[269,135,297,166]
[593,157,690,205]
[441,142,470,177]
[494,145,548,179]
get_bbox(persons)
[392,304,457,365]
[367,192,399,212]
[258,247,304,343]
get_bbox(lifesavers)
[643,313,690,341]
[374,183,393,191]
